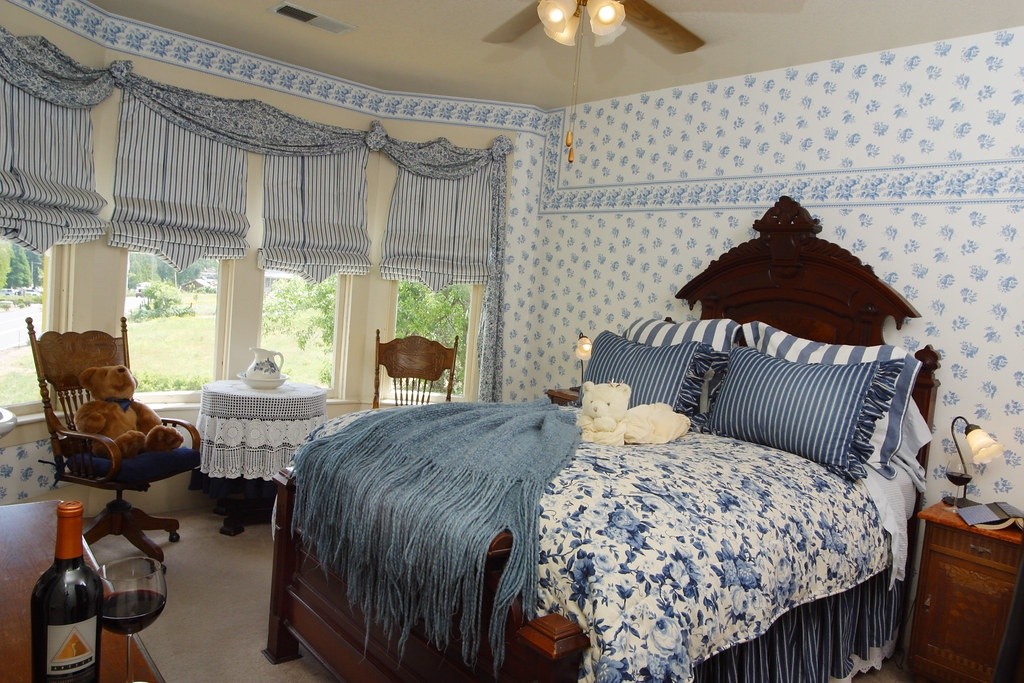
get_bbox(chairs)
[373,328,459,409]
[25,316,201,576]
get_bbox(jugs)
[246,348,284,379]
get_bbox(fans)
[481,0,706,55]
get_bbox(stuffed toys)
[74,365,184,457]
[574,381,689,445]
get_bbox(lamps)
[536,0,627,162]
[941,416,1004,509]
[569,332,592,391]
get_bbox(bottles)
[31,501,102,683]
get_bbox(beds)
[262,194,942,683]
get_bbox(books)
[956,501,1024,531]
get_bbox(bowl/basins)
[237,371,289,389]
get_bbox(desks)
[188,380,327,536]
[0,499,166,683]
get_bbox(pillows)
[621,316,742,414]
[742,320,923,480]
[576,330,715,419]
[692,345,905,483]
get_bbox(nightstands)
[906,496,1024,683]
[545,389,579,406]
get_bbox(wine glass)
[942,455,974,513]
[98,557,167,683]
